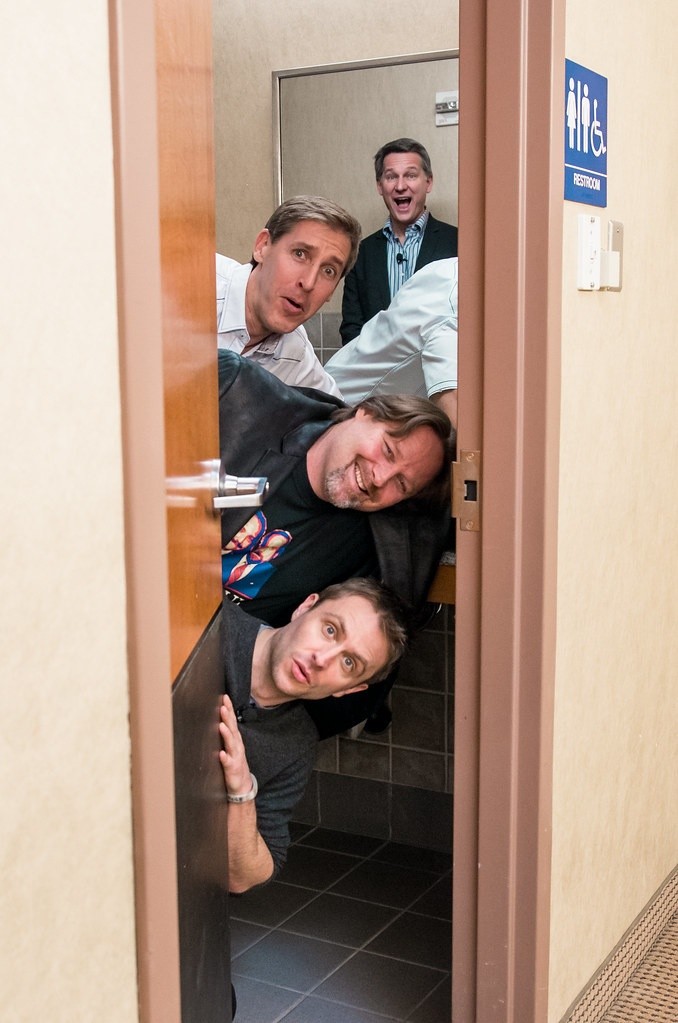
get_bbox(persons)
[216,196,364,399]
[338,138,458,345]
[324,257,458,629]
[217,318,451,627]
[218,578,408,1022]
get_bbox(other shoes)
[361,694,392,735]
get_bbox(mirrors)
[272,48,459,312]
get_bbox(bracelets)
[227,774,258,802]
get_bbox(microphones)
[397,253,403,264]
[236,707,245,724]
